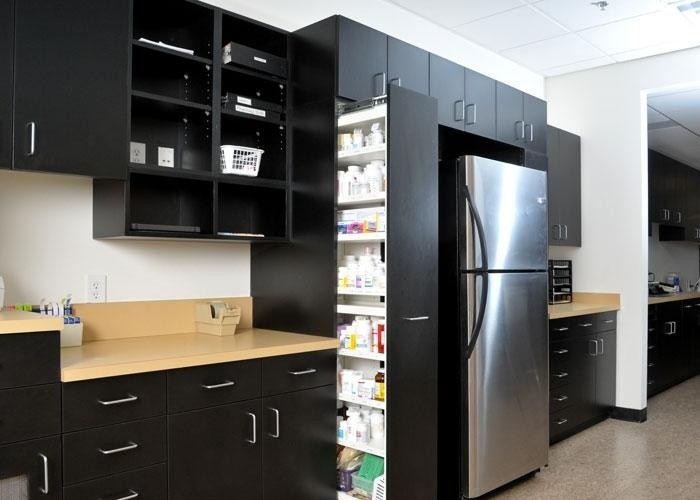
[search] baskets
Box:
[220,144,265,177]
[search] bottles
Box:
[338,121,386,444]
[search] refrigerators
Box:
[438,151,550,500]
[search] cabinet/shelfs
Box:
[549,310,617,447]
[291,11,430,102]
[334,81,445,500]
[428,52,498,142]
[168,358,262,499]
[647,298,700,399]
[523,124,581,247]
[496,82,549,154]
[0,326,60,500]
[63,371,169,500]
[648,148,700,242]
[261,347,339,500]
